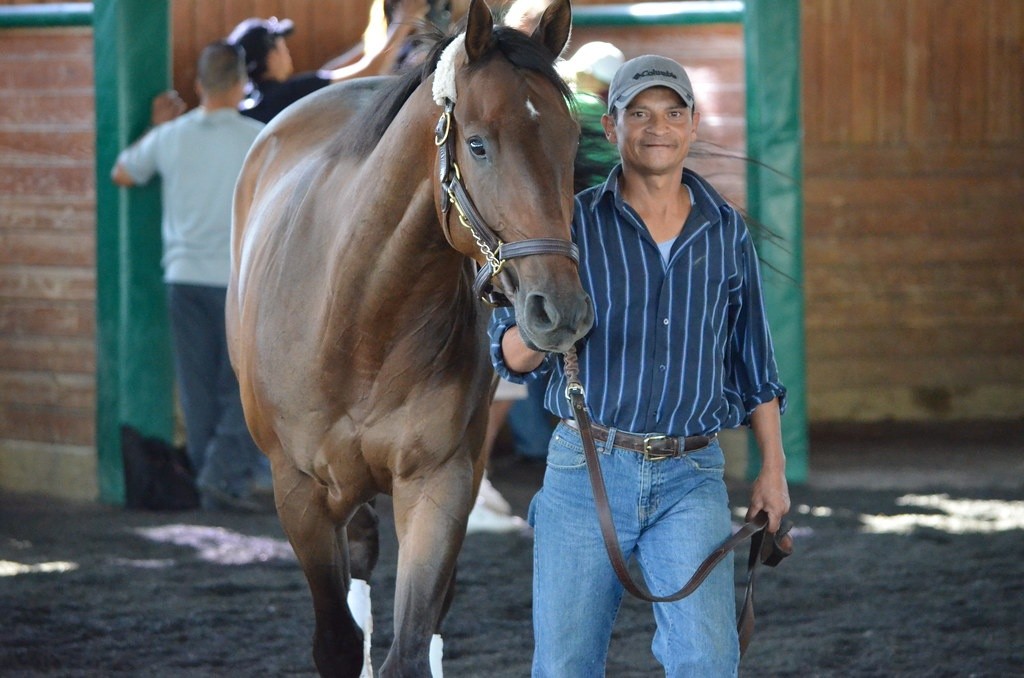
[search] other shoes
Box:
[197,466,276,514]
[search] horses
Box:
[222,0,804,678]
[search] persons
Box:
[488,53,790,676]
[112,39,274,515]
[225,0,391,124]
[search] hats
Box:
[226,17,295,72]
[606,55,695,116]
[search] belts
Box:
[562,417,718,461]
